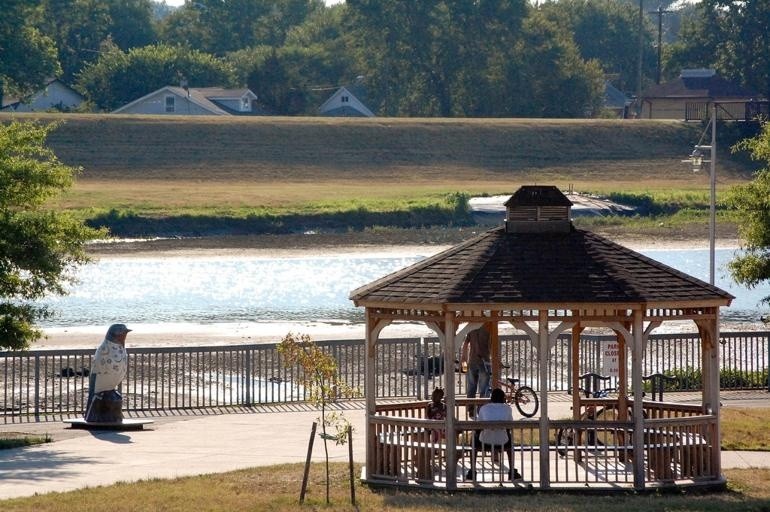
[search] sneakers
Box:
[508,469,522,480]
[465,470,477,480]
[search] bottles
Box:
[462,361,467,372]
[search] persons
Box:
[630,98,642,120]
[461,321,505,419]
[466,389,522,480]
[425,386,465,442]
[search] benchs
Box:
[379,424,706,483]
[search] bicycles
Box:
[465,359,541,419]
[594,391,647,449]
[557,385,618,455]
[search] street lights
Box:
[680,107,718,287]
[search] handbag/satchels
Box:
[483,362,492,378]
